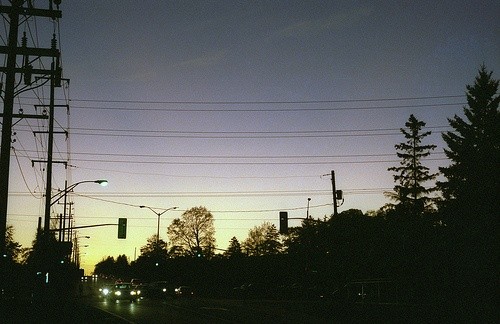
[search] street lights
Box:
[139,204,179,248]
[44,179,107,237]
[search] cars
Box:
[98,279,195,305]
